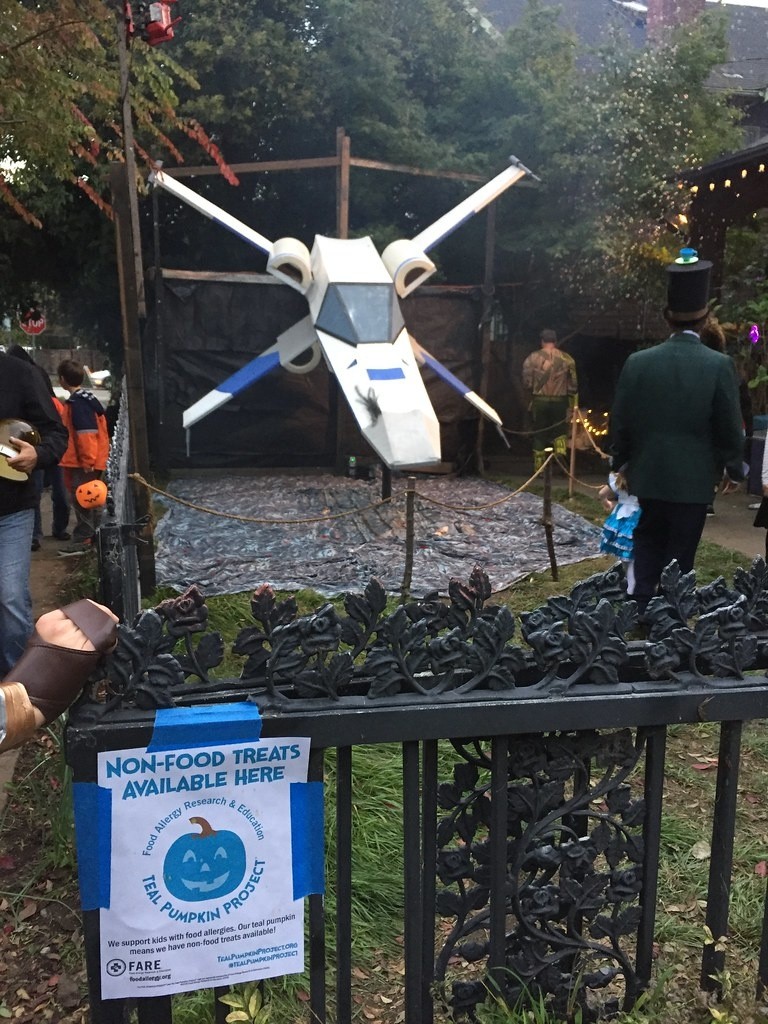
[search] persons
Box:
[0,351,70,679]
[598,464,641,601]
[612,249,746,590]
[57,360,110,554]
[754,428,768,563]
[521,331,577,475]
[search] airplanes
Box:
[148,153,543,473]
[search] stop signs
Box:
[20,309,46,335]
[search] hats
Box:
[662,247,715,321]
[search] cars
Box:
[89,370,112,389]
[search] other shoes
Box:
[706,504,715,516]
[30,539,41,550]
[52,529,71,540]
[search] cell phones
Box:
[0,442,20,458]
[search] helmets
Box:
[0,418,42,481]
